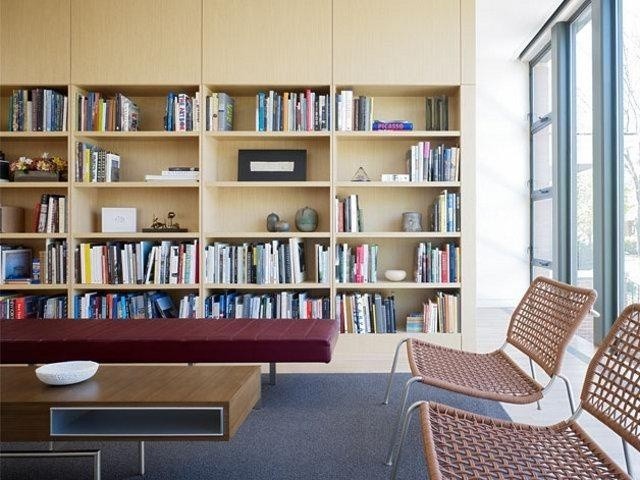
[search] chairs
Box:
[381,274,605,470]
[385,303,640,480]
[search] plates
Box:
[33,360,102,386]
[38,384,102,402]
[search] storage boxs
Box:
[96,205,138,234]
[0,203,27,234]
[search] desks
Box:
[1,366,262,480]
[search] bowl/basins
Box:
[385,269,406,282]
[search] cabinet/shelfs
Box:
[71,84,200,320]
[202,85,332,321]
[0,83,71,319]
[336,86,461,333]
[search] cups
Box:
[402,212,423,230]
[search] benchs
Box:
[0,315,341,387]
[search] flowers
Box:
[8,151,67,174]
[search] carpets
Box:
[0,372,519,480]
